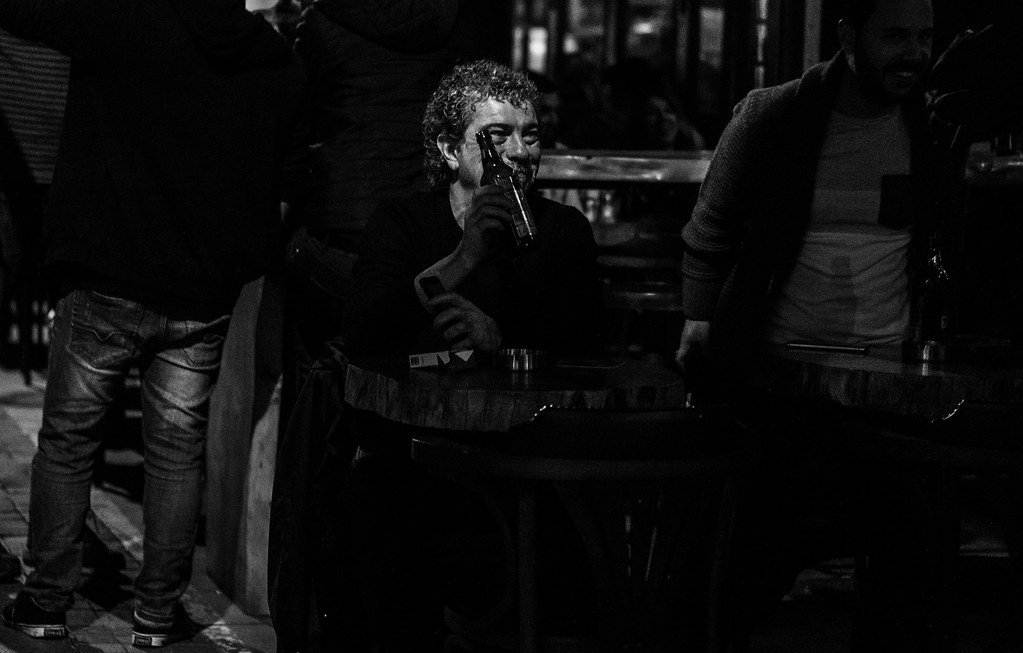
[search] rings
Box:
[465,319,474,331]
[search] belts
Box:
[286,225,345,294]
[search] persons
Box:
[0,0,306,647]
[0,0,1023,653]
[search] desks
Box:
[342,351,759,653]
[753,336,1023,653]
[603,274,708,346]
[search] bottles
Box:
[475,129,540,251]
[909,230,951,363]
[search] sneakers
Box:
[1,590,66,637]
[131,606,174,647]
